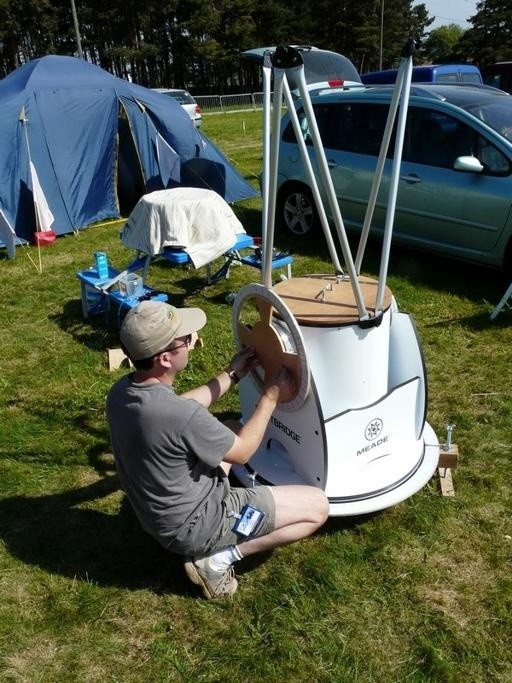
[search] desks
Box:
[119,231,255,286]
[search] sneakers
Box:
[184,551,238,601]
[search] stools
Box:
[108,284,169,329]
[76,264,124,324]
[240,251,293,280]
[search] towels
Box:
[0,209,16,258]
[27,160,55,233]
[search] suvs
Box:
[240,45,512,274]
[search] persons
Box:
[106,300,330,601]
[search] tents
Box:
[0,54,260,248]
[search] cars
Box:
[149,88,202,129]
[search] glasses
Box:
[165,333,192,352]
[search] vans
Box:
[361,63,511,94]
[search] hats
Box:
[120,299,207,361]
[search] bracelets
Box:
[225,367,240,385]
